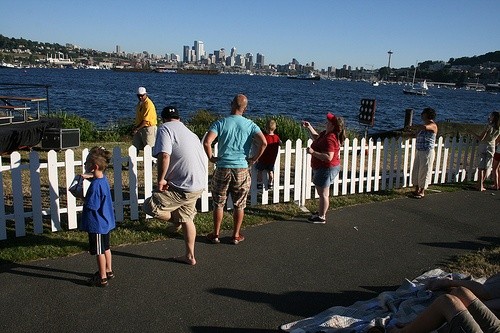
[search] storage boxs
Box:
[42,128,81,152]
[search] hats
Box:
[327,112,341,132]
[136,86,147,94]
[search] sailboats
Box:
[402,59,427,95]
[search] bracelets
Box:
[312,151,315,154]
[135,128,139,131]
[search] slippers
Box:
[414,194,425,198]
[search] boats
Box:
[284,69,321,82]
[154,66,222,76]
[370,80,381,88]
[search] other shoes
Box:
[95,270,114,278]
[207,232,220,243]
[307,214,326,223]
[231,234,244,244]
[89,277,108,286]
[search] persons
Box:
[122,87,158,170]
[468,111,500,192]
[142,106,205,266]
[252,120,282,189]
[402,108,438,198]
[78,146,116,287]
[386,286,500,333]
[204,94,268,245]
[301,114,345,224]
